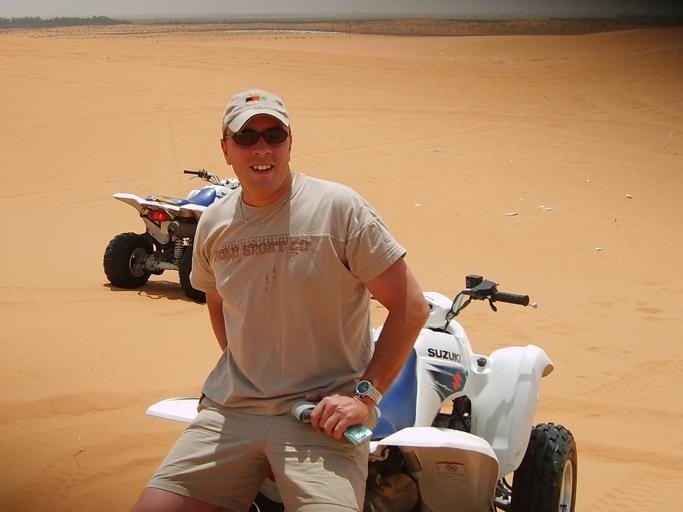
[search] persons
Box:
[128,89,431,510]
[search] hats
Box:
[220,90,290,136]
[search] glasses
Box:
[222,126,287,146]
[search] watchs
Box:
[354,380,383,405]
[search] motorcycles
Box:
[100,167,241,303]
[137,273,581,512]
